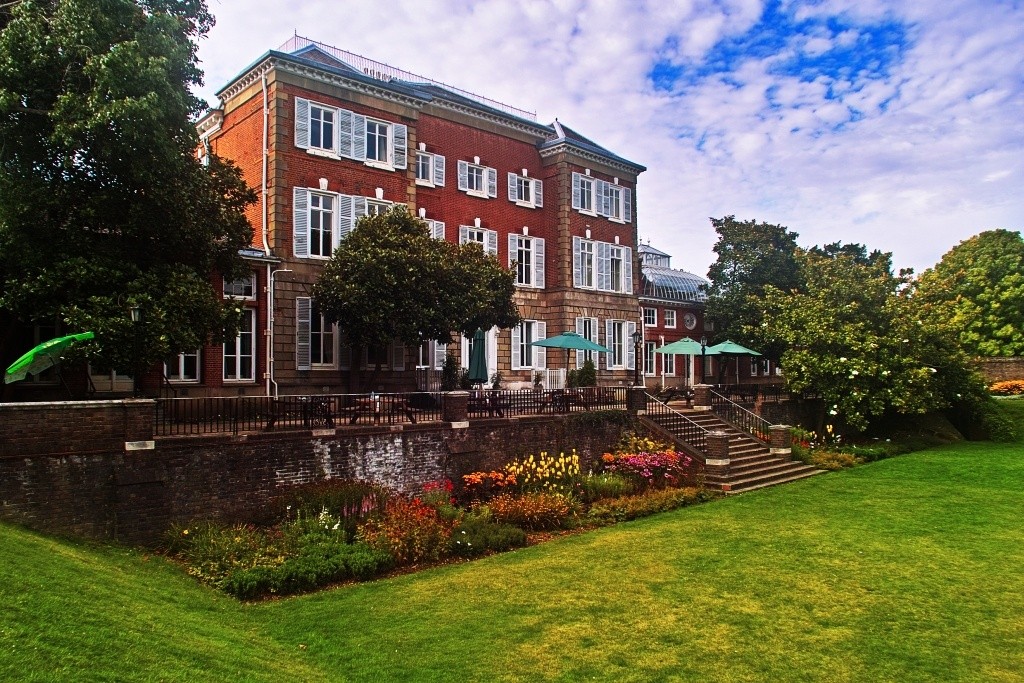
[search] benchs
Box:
[258,411,287,418]
[342,406,371,411]
[534,400,553,404]
[399,406,423,414]
[657,393,672,397]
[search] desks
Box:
[537,392,591,413]
[349,396,417,425]
[661,390,695,408]
[263,400,336,432]
[716,388,752,403]
[468,397,505,418]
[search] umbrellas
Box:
[528,331,612,382]
[650,336,722,389]
[708,339,763,390]
[4,331,97,401]
